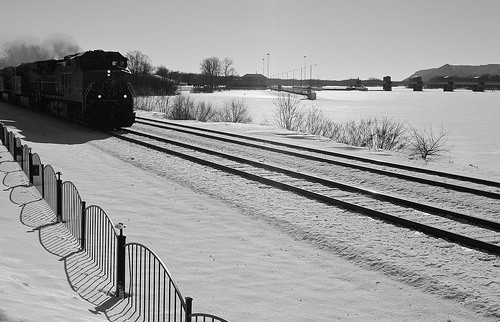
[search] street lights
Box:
[267,53,270,85]
[304,55,306,86]
[262,59,264,75]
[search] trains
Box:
[0,49,136,131]
